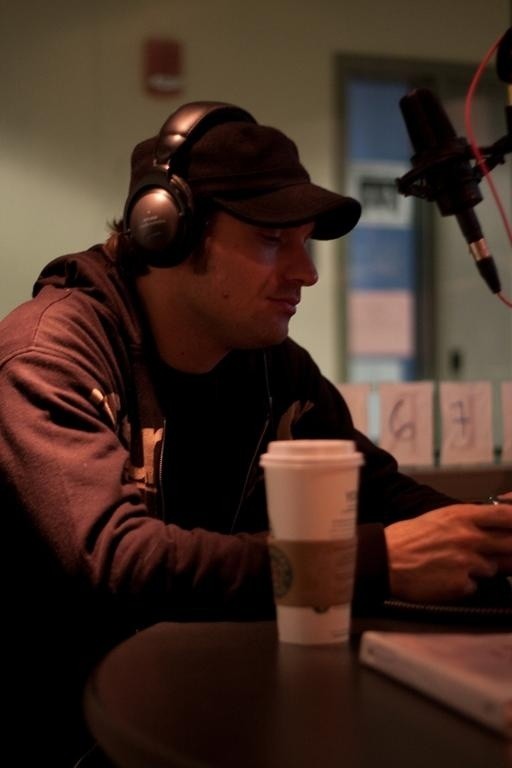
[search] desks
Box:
[81,584,512,768]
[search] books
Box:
[358,627,512,735]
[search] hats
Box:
[128,119,361,241]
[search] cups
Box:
[260,439,367,646]
[274,639,360,767]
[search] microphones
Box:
[399,87,502,293]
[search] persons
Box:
[0,101,512,767]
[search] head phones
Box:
[122,101,256,268]
